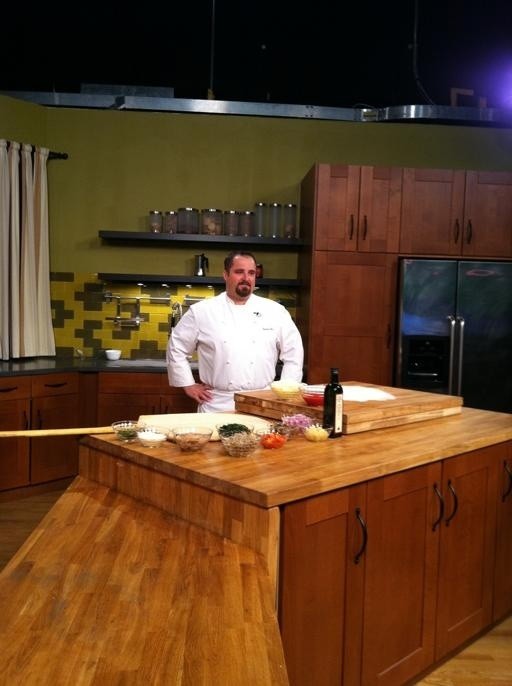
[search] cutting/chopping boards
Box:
[233,378,463,434]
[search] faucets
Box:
[172,301,184,320]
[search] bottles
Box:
[323,367,344,436]
[148,202,298,238]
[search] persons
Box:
[165,248,304,413]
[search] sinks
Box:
[106,357,200,372]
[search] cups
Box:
[194,253,209,276]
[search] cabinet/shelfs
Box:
[269,475,368,686]
[1,365,97,496]
[297,159,401,253]
[492,433,512,628]
[96,227,311,289]
[398,165,510,262]
[92,365,204,431]
[294,249,399,389]
[358,442,501,686]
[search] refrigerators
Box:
[396,255,511,413]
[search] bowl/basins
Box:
[217,421,287,458]
[299,384,326,406]
[299,423,332,442]
[136,425,171,448]
[112,420,148,444]
[268,379,308,400]
[171,426,214,452]
[105,349,122,361]
